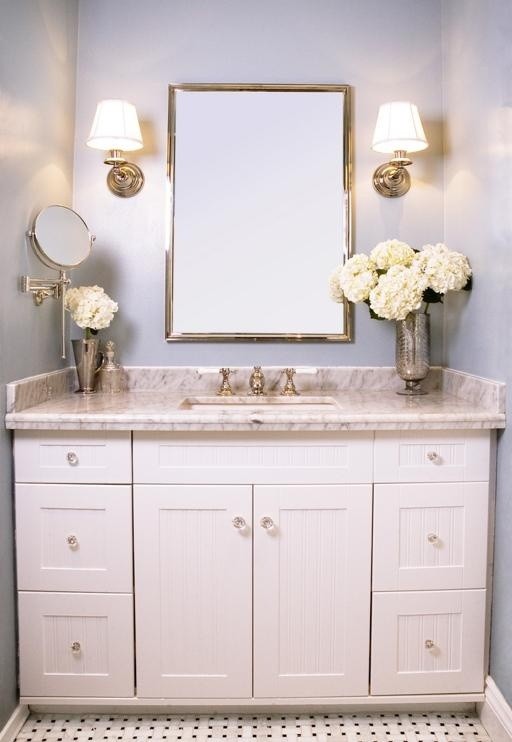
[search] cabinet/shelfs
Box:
[12,431,490,707]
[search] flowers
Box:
[64,285,119,339]
[338,239,472,322]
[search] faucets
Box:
[248,366,266,395]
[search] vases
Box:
[395,301,431,396]
[71,338,103,394]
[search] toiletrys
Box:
[99,341,124,394]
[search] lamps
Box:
[86,99,145,198]
[370,101,429,198]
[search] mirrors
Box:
[32,205,92,267]
[164,83,354,345]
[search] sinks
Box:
[176,395,345,414]
